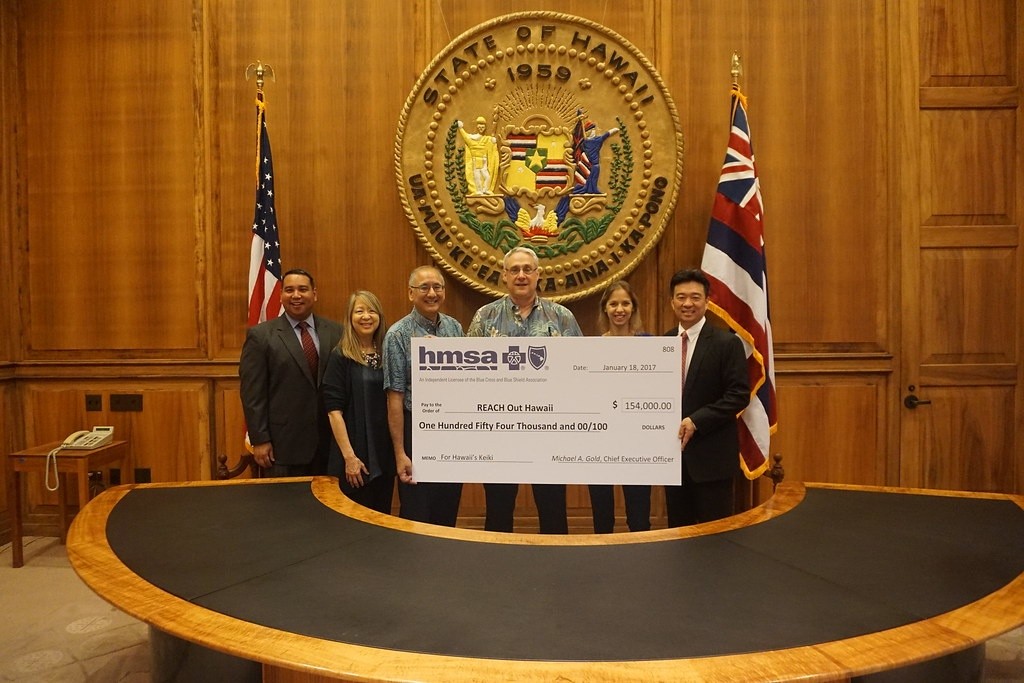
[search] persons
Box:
[588,280,652,534]
[662,268,750,531]
[467,247,583,535]
[382,266,464,528]
[321,290,395,515]
[237,269,344,477]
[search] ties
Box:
[299,322,318,385]
[681,331,688,389]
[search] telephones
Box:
[61,426,114,450]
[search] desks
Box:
[7,440,129,567]
[64,473,1024,683]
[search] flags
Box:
[244,90,286,453]
[700,89,778,480]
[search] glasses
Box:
[506,267,538,275]
[409,284,445,293]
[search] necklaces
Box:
[362,339,381,370]
